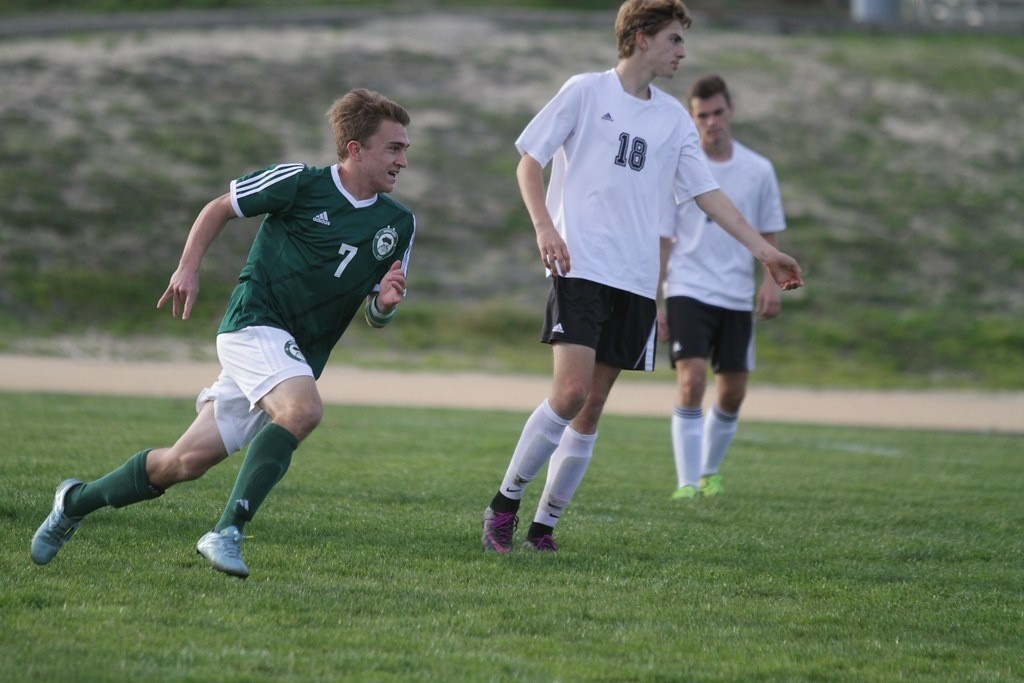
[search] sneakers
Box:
[196,525,250,579]
[481,506,520,555]
[522,535,558,553]
[30,478,87,565]
[700,473,724,498]
[672,485,697,501]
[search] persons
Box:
[654,76,789,500]
[481,1,804,552]
[30,88,418,580]
